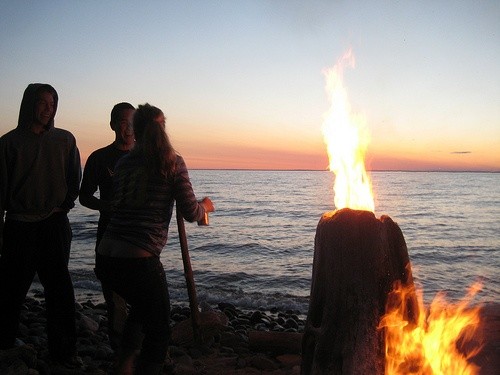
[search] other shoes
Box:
[59,356,83,369]
[161,350,176,372]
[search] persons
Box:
[0,83,82,375]
[78,103,214,375]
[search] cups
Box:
[198,200,209,226]
